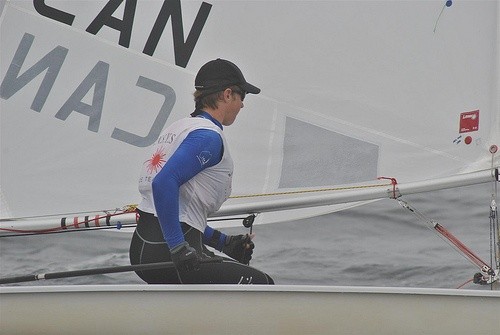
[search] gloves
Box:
[172,240,204,273]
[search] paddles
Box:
[1,257,222,285]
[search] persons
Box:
[129,58,274,287]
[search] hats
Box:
[197,60,262,95]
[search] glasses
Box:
[231,88,247,100]
